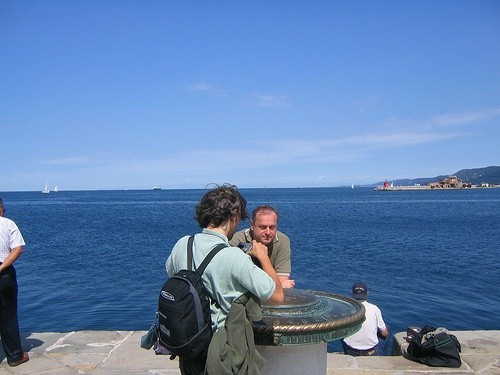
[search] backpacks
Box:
[156,235,228,360]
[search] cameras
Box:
[237,242,252,254]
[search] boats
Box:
[153,186,161,191]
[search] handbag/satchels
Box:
[404,327,462,368]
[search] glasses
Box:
[0,206,6,212]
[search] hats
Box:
[353,283,368,300]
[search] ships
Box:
[374,175,500,191]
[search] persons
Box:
[228,206,295,288]
[165,183,284,375]
[340,282,394,356]
[0,198,29,367]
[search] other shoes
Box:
[9,352,29,365]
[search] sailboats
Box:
[53,186,60,193]
[42,181,49,194]
